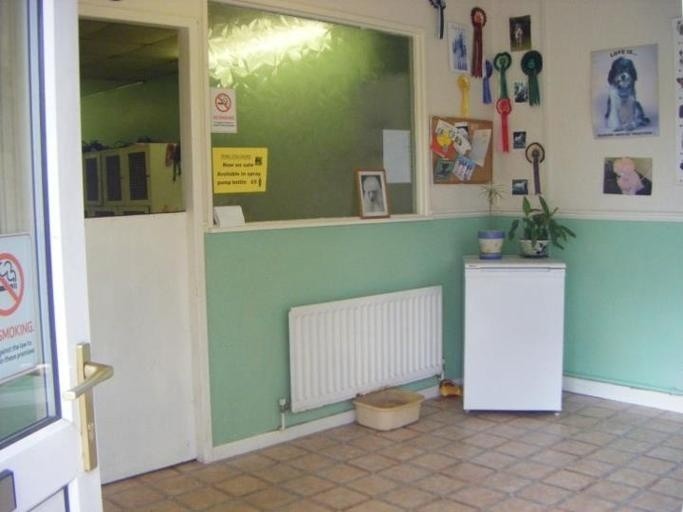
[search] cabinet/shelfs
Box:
[462,254,566,414]
[82,142,185,219]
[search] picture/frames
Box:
[353,168,390,221]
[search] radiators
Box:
[283,282,445,417]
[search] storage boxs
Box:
[352,390,421,429]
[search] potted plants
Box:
[469,182,508,260]
[508,196,575,256]
